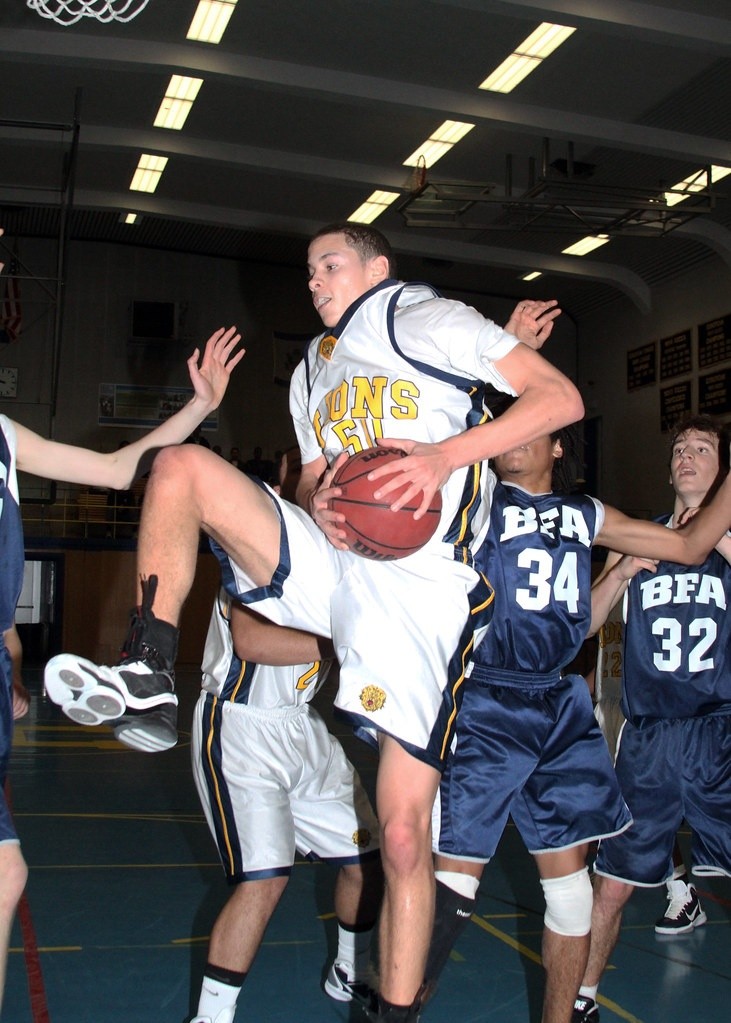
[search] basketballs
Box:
[326,446,443,561]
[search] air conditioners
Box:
[127,299,179,342]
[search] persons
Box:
[572,413,731,1023]
[44,220,588,1023]
[184,445,384,1023]
[422,299,731,1023]
[0,320,248,1023]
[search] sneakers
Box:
[44,572,179,752]
[190,1004,237,1023]
[325,958,380,1012]
[572,994,600,1023]
[654,880,707,935]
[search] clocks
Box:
[0,366,19,399]
